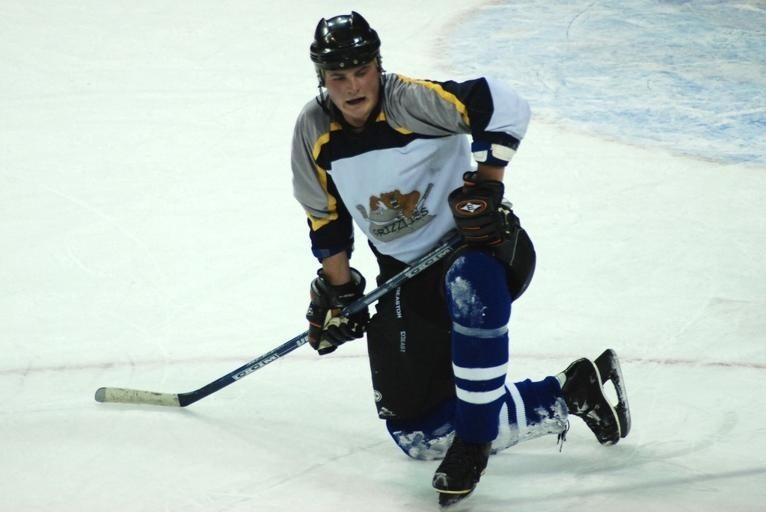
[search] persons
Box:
[290,10,621,496]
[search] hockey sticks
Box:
[94,198,513,407]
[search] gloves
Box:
[447,171,508,249]
[305,267,370,355]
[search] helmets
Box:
[310,11,381,69]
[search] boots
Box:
[431,437,494,495]
[554,358,622,447]
